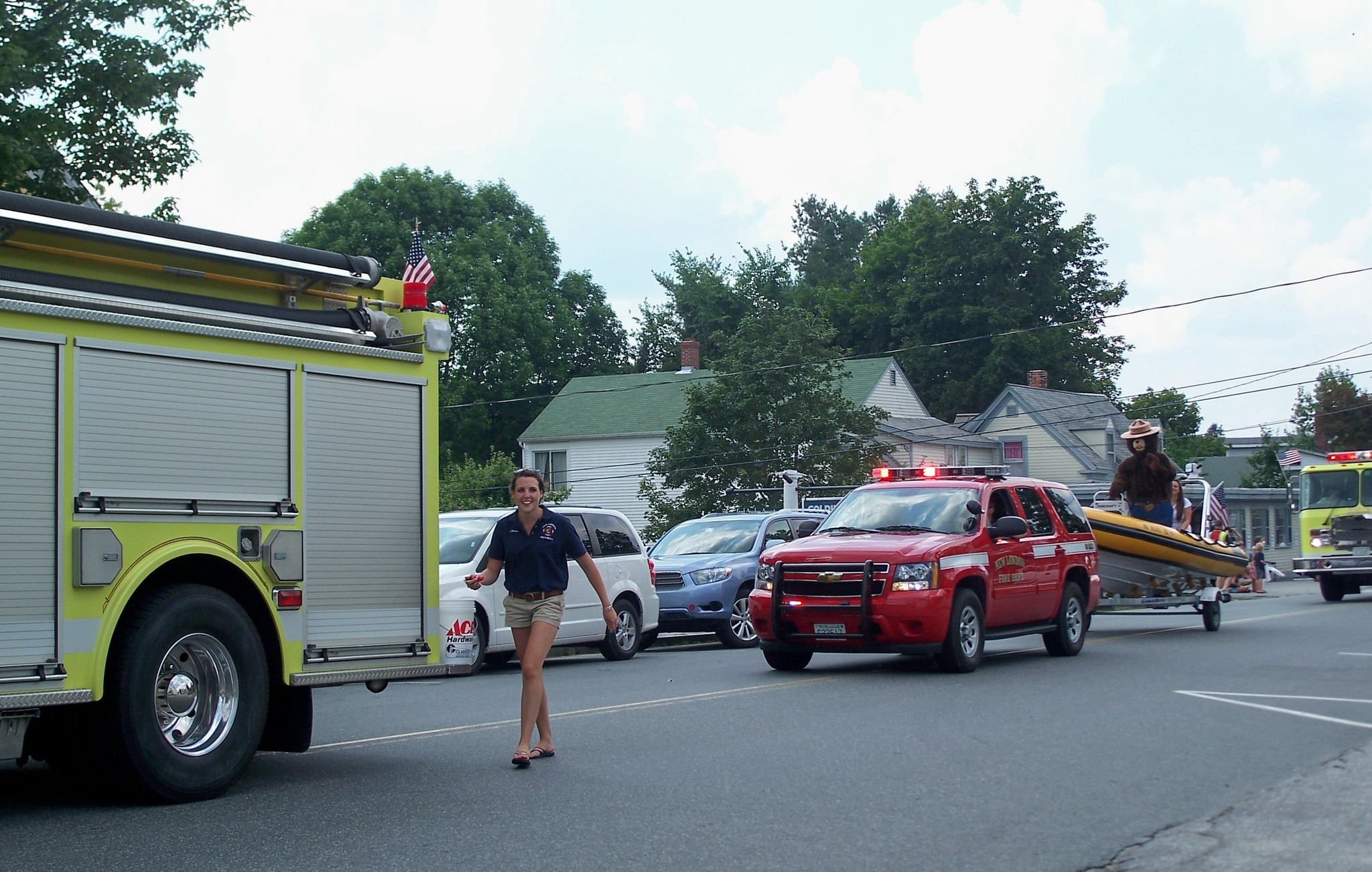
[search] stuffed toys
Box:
[1110,419,1176,529]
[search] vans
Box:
[437,501,660,677]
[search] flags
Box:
[1277,448,1301,466]
[1209,483,1229,530]
[402,227,435,289]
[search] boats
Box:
[1081,463,1249,597]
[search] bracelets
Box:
[601,604,613,611]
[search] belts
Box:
[1131,499,1171,510]
[509,589,563,602]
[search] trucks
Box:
[0,184,451,805]
[1286,450,1372,602]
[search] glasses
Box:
[513,468,540,477]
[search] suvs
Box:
[747,465,1100,672]
[644,509,833,650]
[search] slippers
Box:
[512,750,530,765]
[529,747,555,759]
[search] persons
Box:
[1224,525,1245,588]
[1170,480,1193,534]
[1250,535,1268,594]
[1237,541,1252,580]
[986,498,996,528]
[1209,519,1235,592]
[1315,486,1346,504]
[462,468,620,766]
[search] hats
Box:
[1120,419,1161,439]
[1215,519,1222,526]
